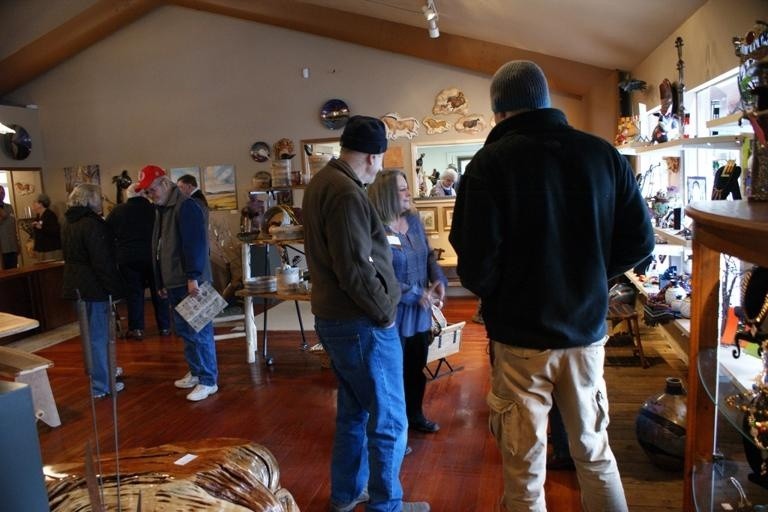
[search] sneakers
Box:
[174,371,200,388]
[186,384,218,401]
[328,483,431,512]
[92,367,125,398]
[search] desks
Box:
[0,312,40,339]
[1,381,50,512]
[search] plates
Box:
[243,275,276,294]
[275,266,299,295]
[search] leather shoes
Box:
[408,415,440,431]
[120,329,171,340]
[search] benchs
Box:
[1,346,62,429]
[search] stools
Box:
[606,302,652,370]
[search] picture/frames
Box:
[415,207,439,235]
[442,207,455,231]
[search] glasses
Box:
[144,179,167,194]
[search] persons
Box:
[61,184,124,399]
[297,114,433,512]
[105,167,210,338]
[134,165,219,401]
[547,396,575,471]
[446,59,656,512]
[0,186,20,270]
[27,194,64,261]
[366,164,448,456]
[415,159,463,199]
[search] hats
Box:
[490,60,550,111]
[340,115,387,154]
[135,165,165,193]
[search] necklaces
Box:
[710,166,735,201]
[724,339,768,480]
[741,270,768,324]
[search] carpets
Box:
[39,437,301,512]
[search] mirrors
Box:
[300,136,341,186]
[409,139,486,204]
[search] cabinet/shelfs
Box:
[0,262,81,352]
[240,206,332,370]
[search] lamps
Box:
[422,0,442,39]
